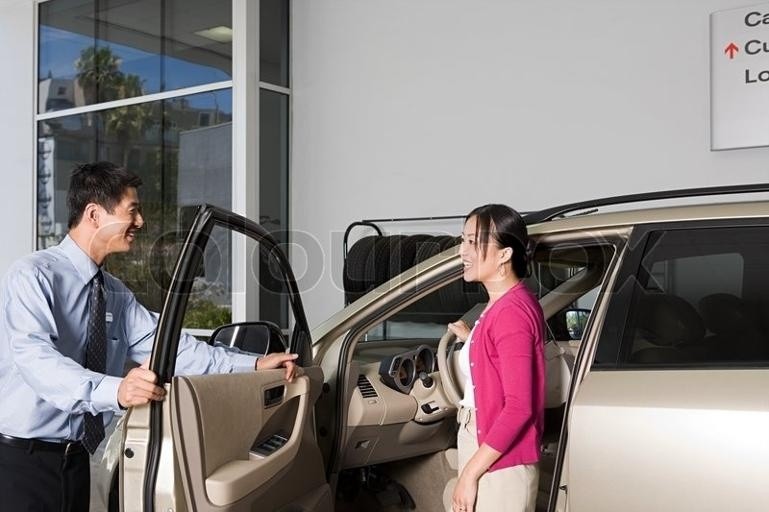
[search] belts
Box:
[457,406,477,424]
[0,433,85,456]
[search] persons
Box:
[448,204,547,512]
[0,160,301,512]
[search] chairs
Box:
[698,293,769,363]
[536,294,707,512]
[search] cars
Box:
[98,183,769,512]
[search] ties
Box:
[80,270,105,455]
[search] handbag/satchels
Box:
[543,319,572,409]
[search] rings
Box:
[458,505,466,511]
